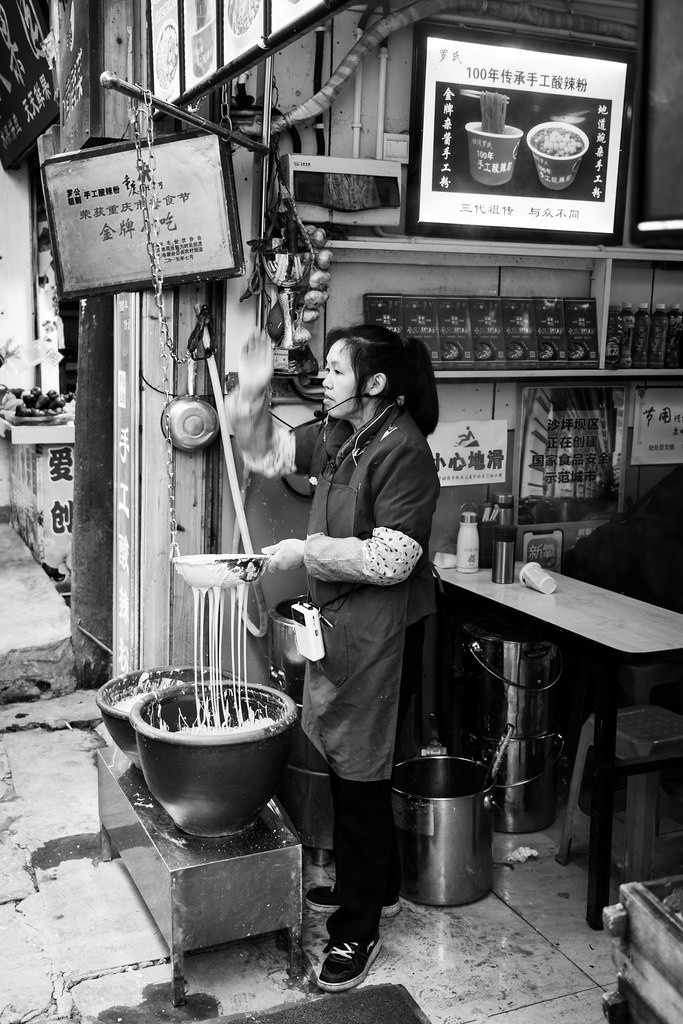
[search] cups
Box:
[519,562,558,594]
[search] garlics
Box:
[293,224,333,344]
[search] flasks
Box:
[492,524,518,584]
[494,493,514,526]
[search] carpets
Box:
[187,982,435,1024]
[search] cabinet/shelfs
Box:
[321,240,683,382]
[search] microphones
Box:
[314,393,372,419]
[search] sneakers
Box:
[305,885,401,918]
[317,931,382,993]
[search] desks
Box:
[428,560,683,930]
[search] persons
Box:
[229,325,439,991]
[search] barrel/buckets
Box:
[456,619,570,834]
[392,754,496,907]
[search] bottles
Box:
[606,300,683,372]
[455,511,479,573]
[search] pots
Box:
[159,345,221,451]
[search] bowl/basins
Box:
[526,121,589,190]
[465,121,524,186]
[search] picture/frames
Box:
[404,18,639,246]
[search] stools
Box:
[555,664,683,884]
